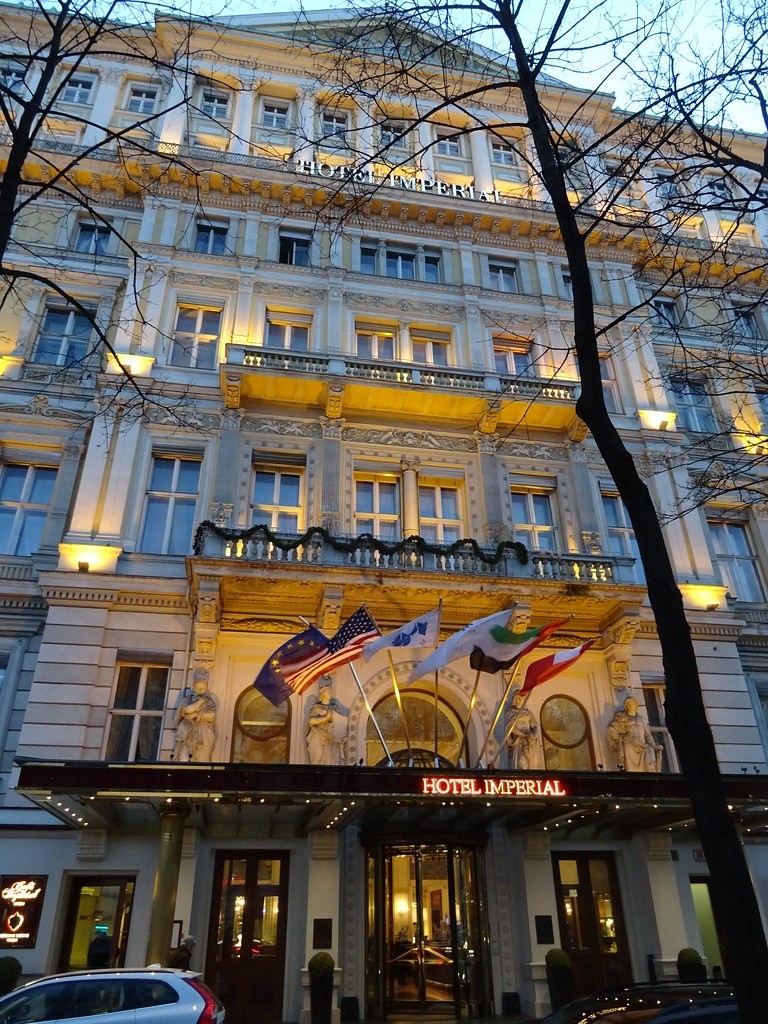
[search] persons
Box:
[169,934,197,970]
[606,696,665,772]
[305,684,348,764]
[86,929,120,970]
[504,689,539,770]
[394,925,412,986]
[170,677,217,763]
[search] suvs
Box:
[0,967,225,1024]
[537,980,740,1024]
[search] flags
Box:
[252,624,330,706]
[517,634,603,697]
[408,609,514,685]
[468,617,570,676]
[361,609,439,664]
[278,604,383,696]
